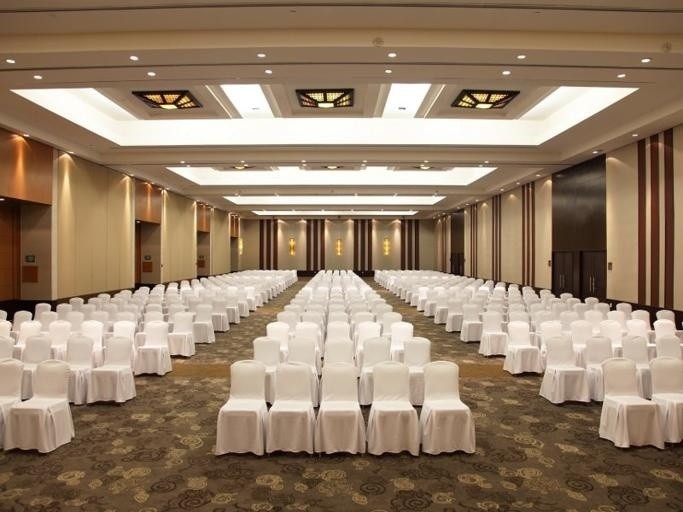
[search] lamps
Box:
[382,237,391,257]
[295,88,354,109]
[335,238,342,256]
[288,238,296,257]
[131,90,203,109]
[450,89,520,109]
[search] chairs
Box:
[216,268,476,456]
[373,269,683,457]
[1,268,299,455]
